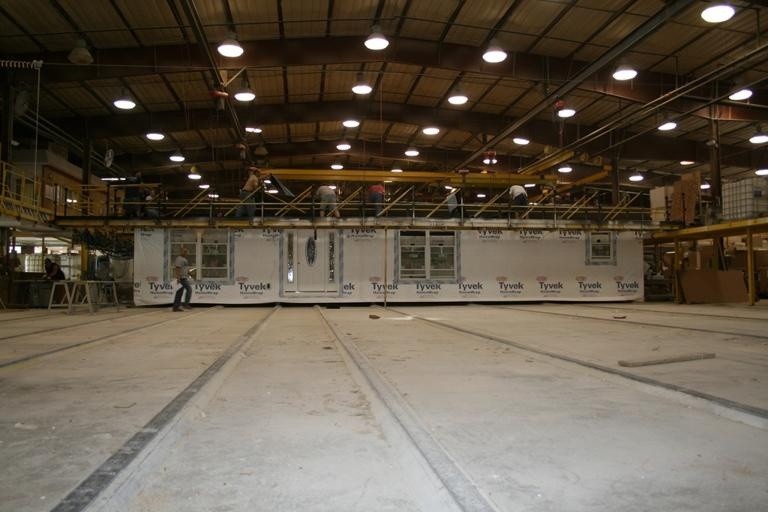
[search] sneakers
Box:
[173,304,191,311]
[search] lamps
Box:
[216,31,263,136]
[513,54,639,147]
[329,23,390,171]
[404,36,508,158]
[113,85,220,200]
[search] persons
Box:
[171,247,200,311]
[316,183,340,217]
[368,182,386,217]
[44,257,65,303]
[509,184,529,219]
[235,169,264,219]
